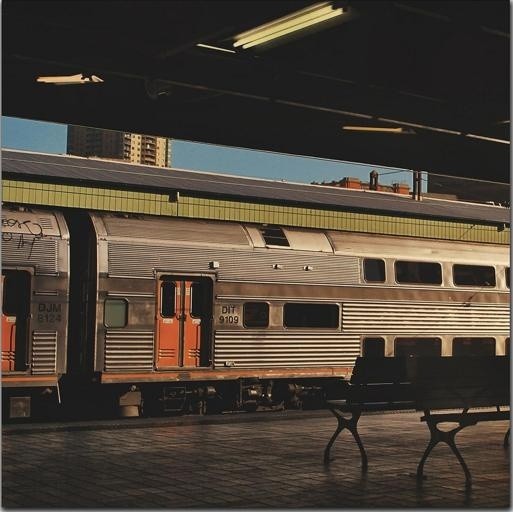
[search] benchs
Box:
[322,356,414,473]
[414,356,511,487]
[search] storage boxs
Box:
[341,125,418,135]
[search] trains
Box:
[1,147,509,418]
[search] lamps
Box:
[232,1,346,50]
[32,74,105,87]
